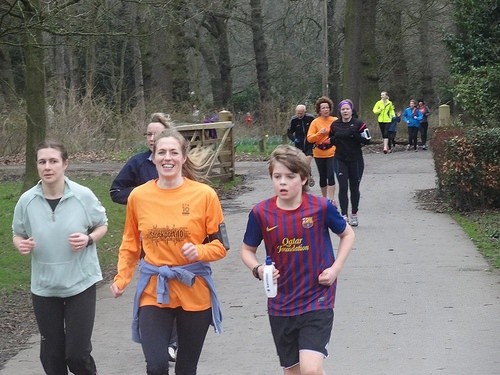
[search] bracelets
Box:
[86,234,93,247]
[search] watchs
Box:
[252,263,262,280]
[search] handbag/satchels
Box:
[314,143,335,150]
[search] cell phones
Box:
[219,223,229,250]
[364,129,371,140]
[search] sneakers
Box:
[348,211,359,226]
[341,215,349,223]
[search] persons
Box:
[12,141,108,375]
[240,143,355,375]
[307,96,339,206]
[372,91,402,154]
[110,113,212,362]
[417,99,432,150]
[244,112,254,128]
[192,104,200,116]
[286,104,316,192]
[402,100,424,152]
[328,99,372,227]
[110,129,230,375]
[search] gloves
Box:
[294,138,301,143]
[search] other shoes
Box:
[388,149,391,153]
[331,200,335,205]
[168,343,178,362]
[383,149,387,154]
[422,144,426,150]
[414,146,418,152]
